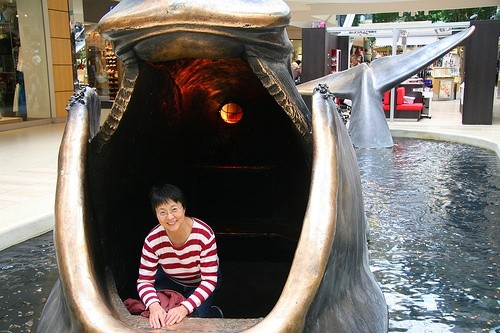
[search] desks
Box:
[399,83,433,118]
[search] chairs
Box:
[396,86,424,120]
[381,90,390,116]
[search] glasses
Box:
[156,206,183,215]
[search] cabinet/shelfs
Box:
[331,49,341,107]
[97,48,120,100]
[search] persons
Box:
[138,182,219,329]
